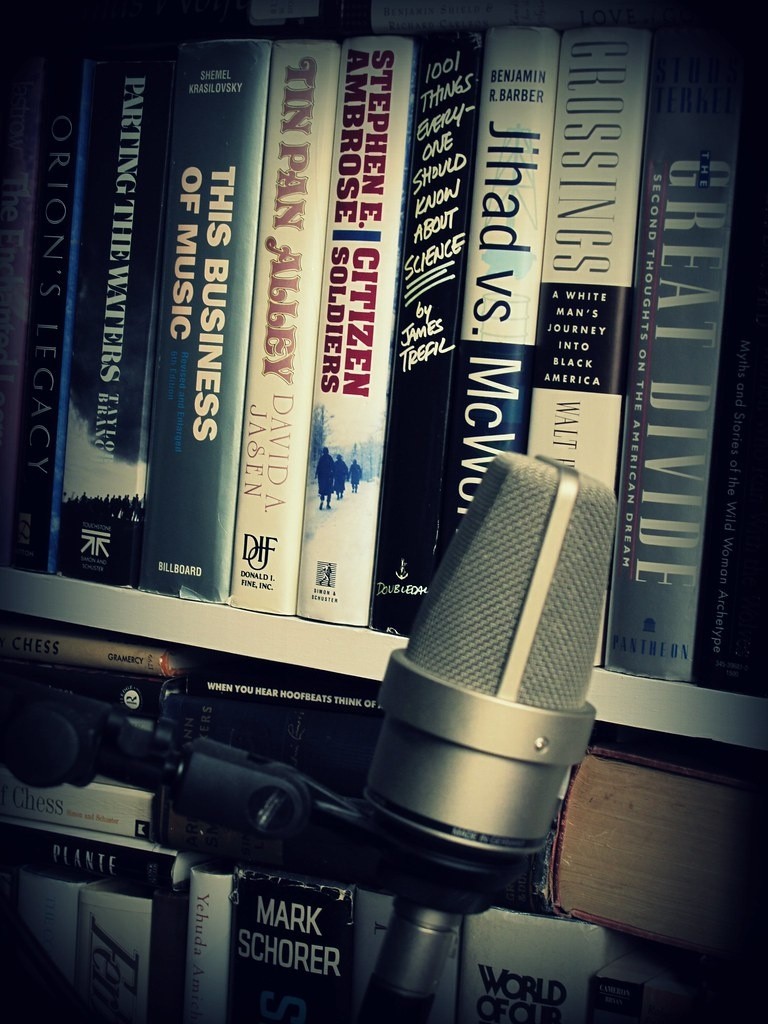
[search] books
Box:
[0,0,768,1024]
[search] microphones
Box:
[354,450,616,1024]
